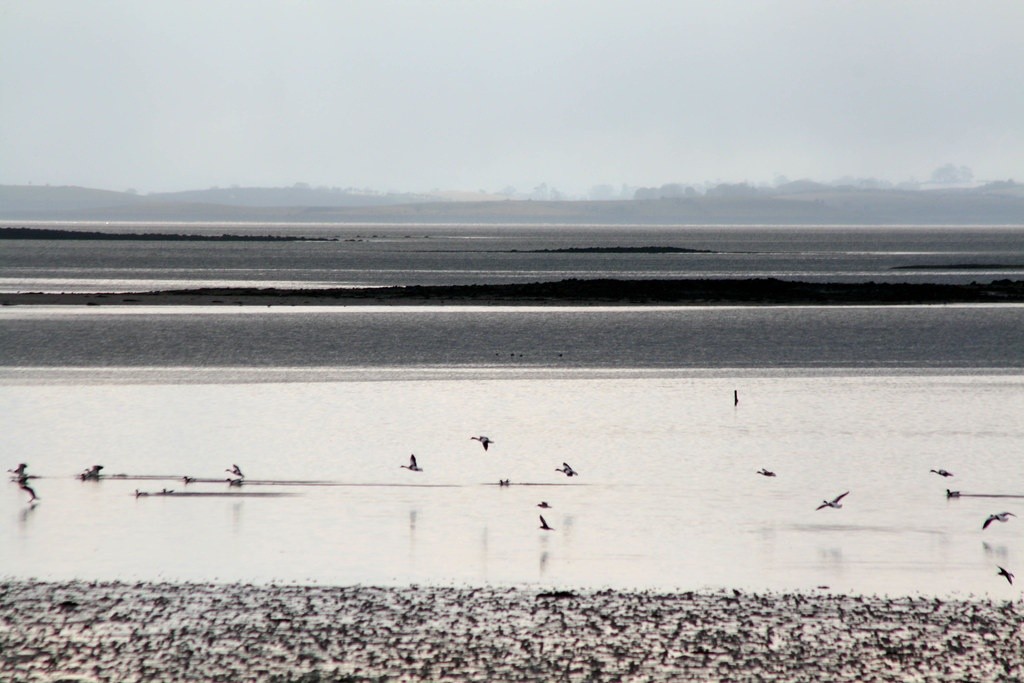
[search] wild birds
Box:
[183,476,195,484]
[997,565,1014,585]
[471,436,494,451]
[816,491,849,511]
[135,488,174,498]
[81,466,103,481]
[7,463,39,502]
[946,488,960,497]
[757,467,775,477]
[537,501,553,529]
[555,462,579,477]
[930,469,952,477]
[225,464,245,486]
[401,454,422,471]
[983,512,1018,529]
